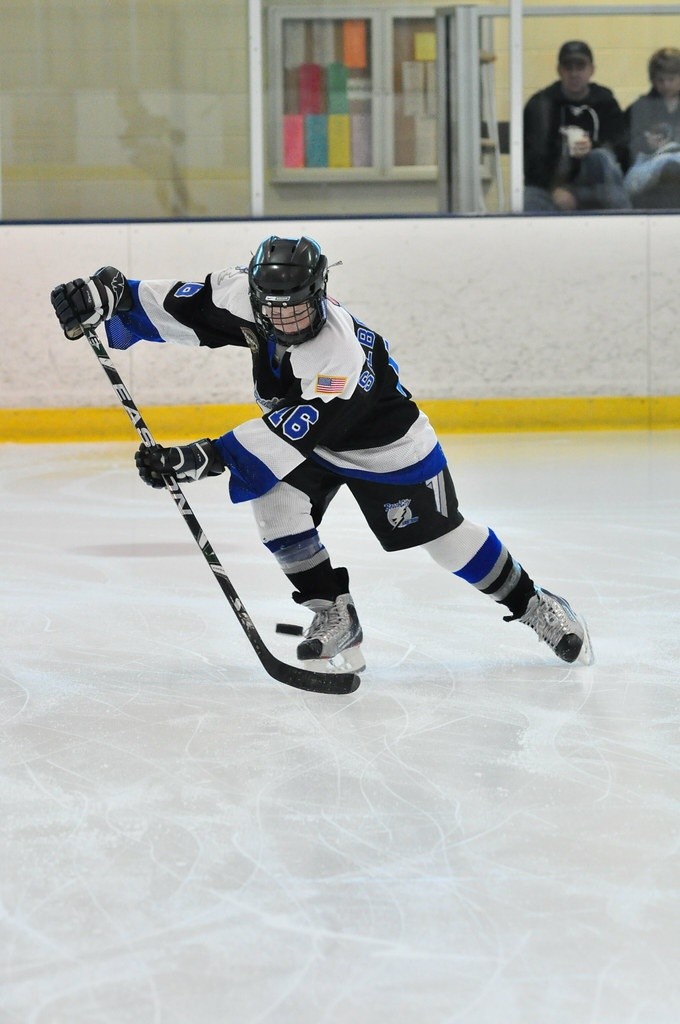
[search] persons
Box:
[51,236,596,675]
[524,41,680,211]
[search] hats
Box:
[559,42,593,64]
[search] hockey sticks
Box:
[80,322,362,695]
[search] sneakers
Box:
[496,563,594,668]
[275,566,367,674]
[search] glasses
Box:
[561,62,588,71]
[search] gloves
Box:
[133,436,226,489]
[49,267,132,341]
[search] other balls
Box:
[275,623,303,636]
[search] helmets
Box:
[248,236,329,344]
